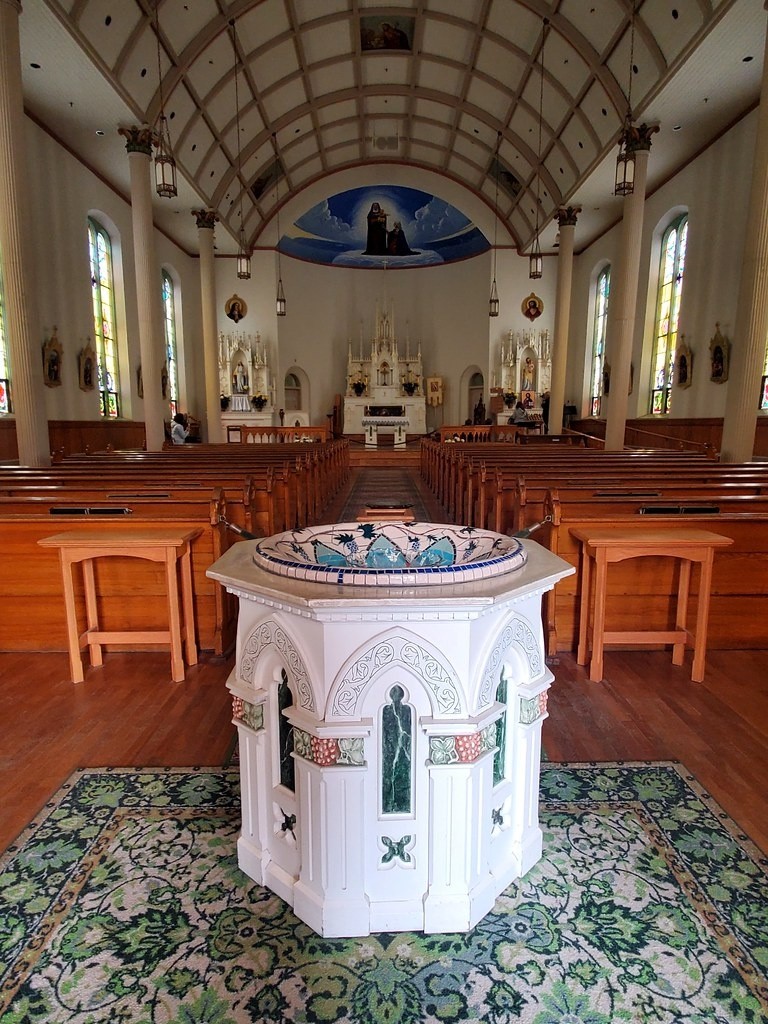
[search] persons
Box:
[366,203,420,256]
[229,303,243,320]
[523,393,533,409]
[524,357,535,391]
[542,392,566,429]
[171,412,190,444]
[508,402,528,424]
[233,360,246,393]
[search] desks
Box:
[36,527,205,683]
[361,416,410,449]
[568,527,734,683]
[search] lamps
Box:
[154,0,286,317]
[489,0,636,317]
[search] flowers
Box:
[402,382,418,392]
[250,395,268,408]
[353,382,367,394]
[220,392,233,409]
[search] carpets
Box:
[0,760,768,1023]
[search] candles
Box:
[402,404,404,410]
[367,404,369,410]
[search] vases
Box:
[357,392,361,396]
[221,408,226,412]
[408,392,413,396]
[256,408,262,412]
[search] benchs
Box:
[419,436,768,657]
[0,439,350,658]
